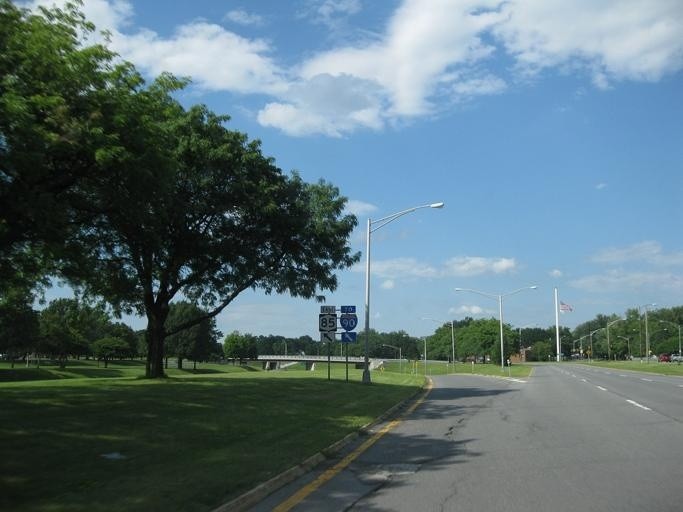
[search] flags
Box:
[560,301,573,312]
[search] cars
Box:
[465,355,492,364]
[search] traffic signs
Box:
[340,314,358,330]
[321,305,336,314]
[341,305,357,314]
[318,314,338,332]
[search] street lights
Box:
[381,343,403,377]
[402,333,428,377]
[453,285,538,370]
[422,316,457,375]
[557,302,683,366]
[362,202,450,384]
